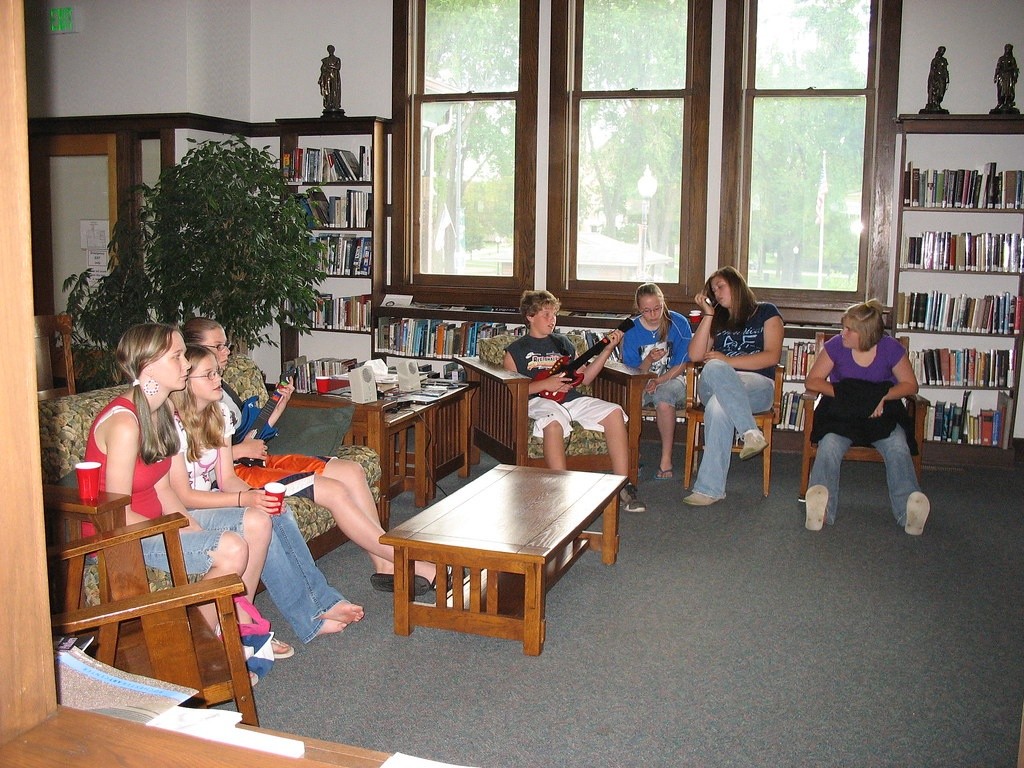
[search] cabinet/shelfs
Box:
[891,114,1024,469]
[274,116,389,399]
[372,308,817,455]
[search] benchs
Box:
[35,355,380,612]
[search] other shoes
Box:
[805,485,828,532]
[370,574,430,595]
[430,566,454,591]
[740,433,769,460]
[683,494,722,505]
[905,492,930,536]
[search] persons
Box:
[994,44,1019,109]
[928,46,949,108]
[169,343,365,644]
[622,283,692,480]
[82,323,295,686]
[504,290,646,512]
[683,265,784,505]
[183,317,454,596]
[320,45,341,110]
[805,298,930,535]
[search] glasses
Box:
[203,342,230,350]
[187,369,223,380]
[639,305,663,314]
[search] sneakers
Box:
[619,482,645,512]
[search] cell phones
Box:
[705,298,712,306]
[657,348,664,351]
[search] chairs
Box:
[34,314,75,404]
[45,511,259,728]
[640,361,786,498]
[798,331,930,503]
[450,332,659,489]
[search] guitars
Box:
[232,364,298,468]
[532,317,636,404]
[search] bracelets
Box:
[238,491,242,507]
[704,314,714,316]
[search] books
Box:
[375,317,526,359]
[903,230,1024,273]
[418,362,465,381]
[283,293,371,332]
[895,336,1016,388]
[903,161,1024,210]
[780,342,815,381]
[776,390,805,431]
[283,146,371,182]
[294,187,372,228]
[308,233,371,275]
[284,356,365,393]
[924,390,1014,450]
[568,329,620,362]
[896,292,1022,334]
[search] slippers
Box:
[655,464,674,479]
[270,637,294,658]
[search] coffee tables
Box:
[377,464,628,656]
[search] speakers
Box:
[396,361,421,392]
[349,366,377,404]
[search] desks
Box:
[233,721,398,768]
[318,379,479,508]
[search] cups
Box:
[690,310,701,321]
[689,313,700,322]
[264,483,286,514]
[74,462,102,500]
[315,376,330,394]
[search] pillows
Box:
[265,405,356,457]
